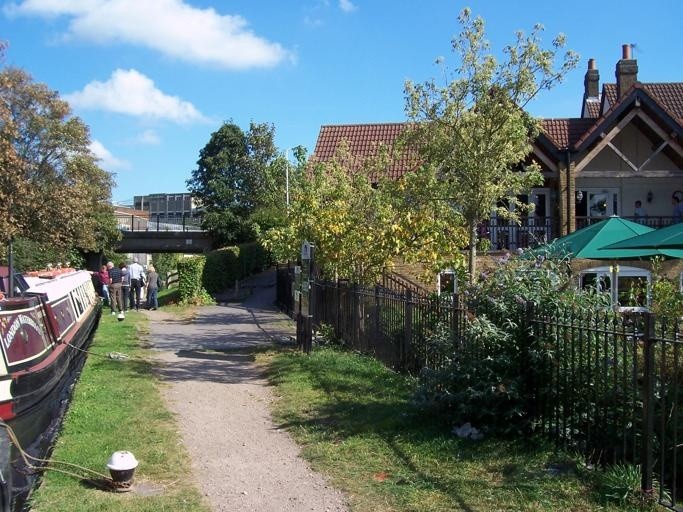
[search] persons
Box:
[86,257,160,316]
[670,196,682,224]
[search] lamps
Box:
[576,190,583,203]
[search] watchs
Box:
[633,199,649,224]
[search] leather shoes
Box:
[124,307,157,311]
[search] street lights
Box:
[284,143,305,215]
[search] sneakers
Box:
[112,312,115,316]
[120,311,124,314]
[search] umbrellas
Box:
[506,216,682,314]
[597,222,682,251]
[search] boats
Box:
[0,233,107,426]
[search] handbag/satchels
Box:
[140,277,145,288]
[157,277,163,289]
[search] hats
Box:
[146,266,155,272]
[132,257,138,262]
